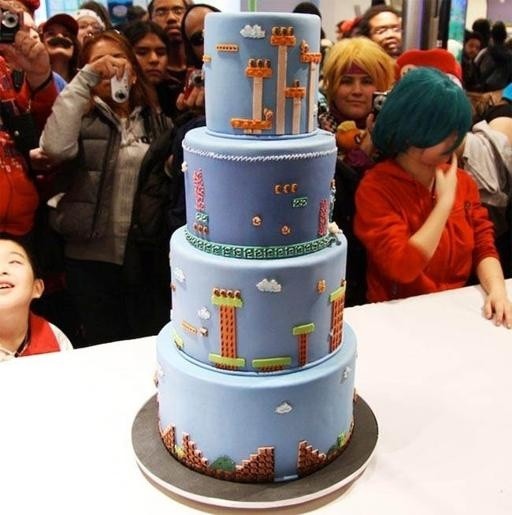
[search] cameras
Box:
[111,66,130,103]
[184,67,204,101]
[0,8,24,43]
[371,92,388,121]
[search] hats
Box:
[393,47,462,83]
[43,14,78,37]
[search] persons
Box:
[0,0,512,362]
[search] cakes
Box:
[154,11,359,485]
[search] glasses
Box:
[186,33,203,47]
[152,7,185,15]
